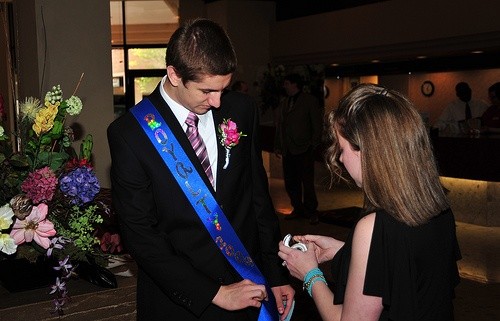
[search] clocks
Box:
[422,81,434,96]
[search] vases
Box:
[0,254,61,294]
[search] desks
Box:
[0,260,137,321]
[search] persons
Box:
[277,85,462,321]
[481,81,500,133]
[432,83,488,129]
[231,80,251,97]
[280,73,322,222]
[107,20,296,321]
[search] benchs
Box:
[439,177,500,285]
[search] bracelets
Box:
[302,267,327,296]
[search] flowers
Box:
[0,72,128,314]
[218,117,247,169]
